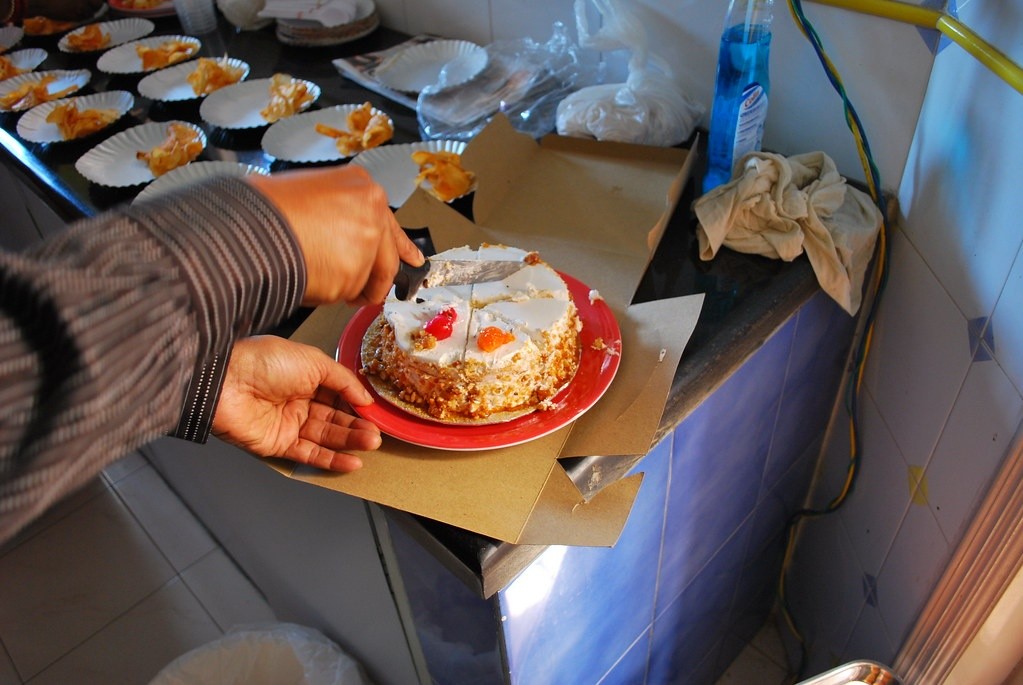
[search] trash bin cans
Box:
[795,659,905,685]
[150,629,364,685]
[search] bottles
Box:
[702,0,775,197]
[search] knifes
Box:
[394,256,532,302]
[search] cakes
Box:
[359,242,584,421]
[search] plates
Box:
[376,40,488,91]
[347,139,476,209]
[58,17,154,54]
[3,48,48,70]
[75,120,206,188]
[108,0,176,17]
[276,0,380,46]
[16,90,135,144]
[334,270,622,451]
[0,69,91,113]
[0,27,24,52]
[96,35,201,73]
[198,76,321,130]
[261,103,394,162]
[130,160,272,207]
[137,58,251,102]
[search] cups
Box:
[172,0,217,36]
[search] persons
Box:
[0,165,425,547]
